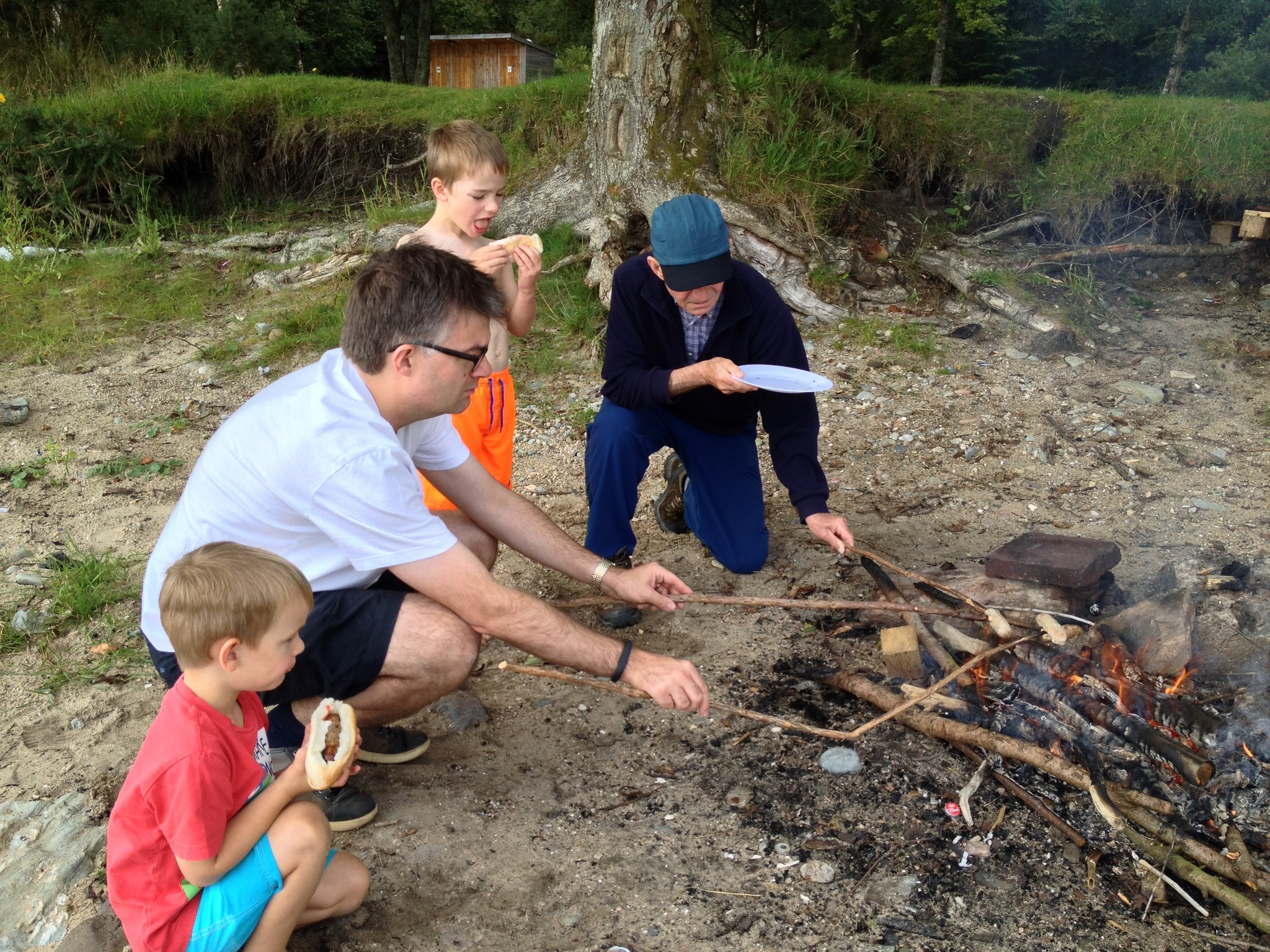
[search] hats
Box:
[651,195,734,291]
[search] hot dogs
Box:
[1041,624,1082,642]
[1036,613,1066,646]
[489,233,544,262]
[984,608,1012,638]
[305,697,356,791]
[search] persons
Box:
[140,243,710,831]
[107,541,370,952]
[395,120,542,640]
[584,194,855,629]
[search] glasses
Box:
[387,341,488,373]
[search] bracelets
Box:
[591,557,616,589]
[611,639,633,682]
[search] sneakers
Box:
[349,721,430,763]
[654,453,690,534]
[266,746,381,830]
[595,547,643,629]
